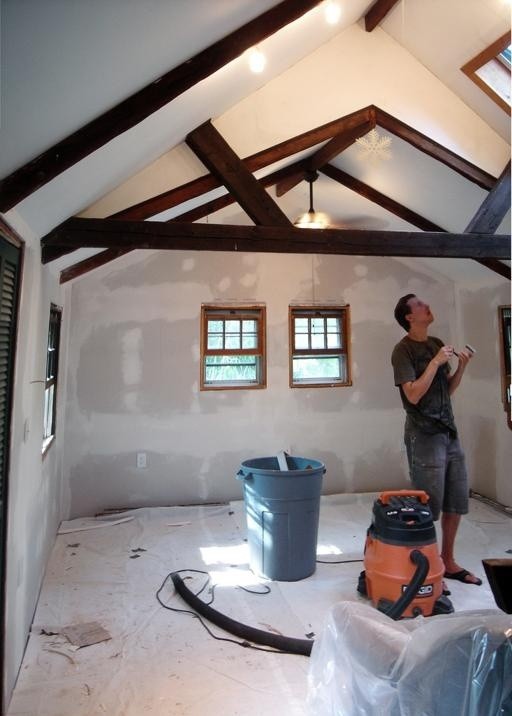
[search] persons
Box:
[392,294,482,587]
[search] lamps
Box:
[295,170,334,229]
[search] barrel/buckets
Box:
[234,455,328,583]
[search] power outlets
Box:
[136,453,146,469]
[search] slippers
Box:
[443,569,482,586]
[442,581,451,596]
